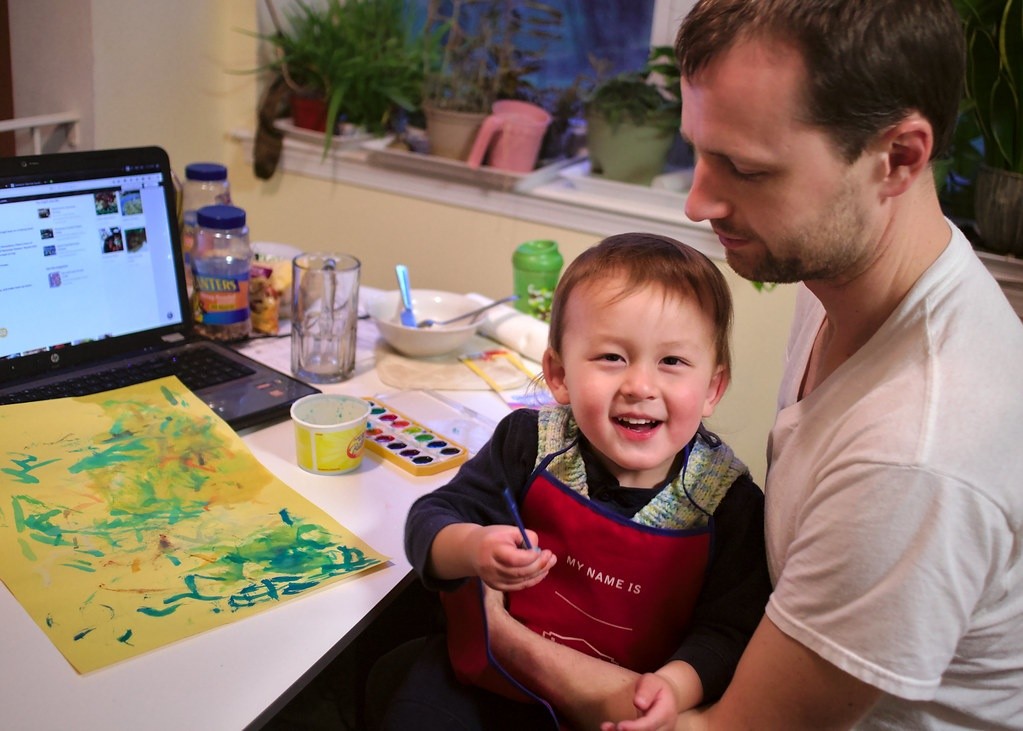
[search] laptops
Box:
[0,146,324,431]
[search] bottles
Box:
[188,205,252,342]
[181,164,233,291]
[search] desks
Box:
[0,282,543,731]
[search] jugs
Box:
[467,99,554,174]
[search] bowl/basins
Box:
[366,289,487,357]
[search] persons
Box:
[478,0,1023,731]
[395,231,771,731]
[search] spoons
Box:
[418,294,521,328]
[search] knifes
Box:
[396,264,417,327]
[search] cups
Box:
[290,392,372,473]
[289,251,362,384]
[511,239,563,323]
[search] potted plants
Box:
[217,0,1023,259]
